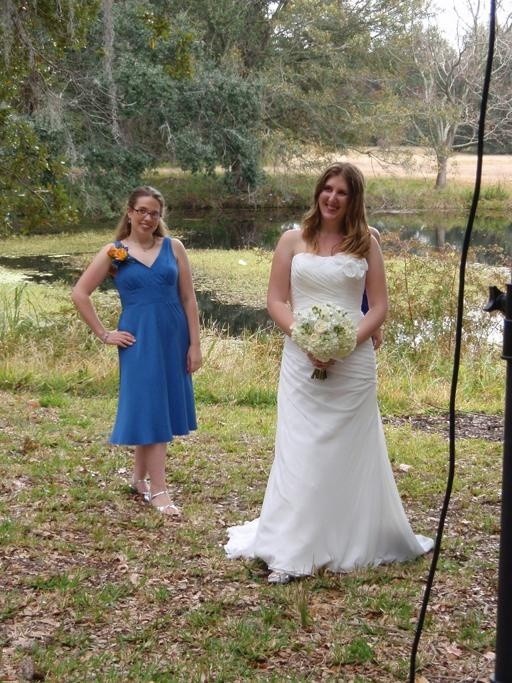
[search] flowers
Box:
[106,244,130,264]
[289,302,358,380]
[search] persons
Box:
[361,226,386,351]
[71,185,203,522]
[224,160,435,585]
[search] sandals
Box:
[149,490,181,520]
[129,473,150,502]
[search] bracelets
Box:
[102,331,110,345]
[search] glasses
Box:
[131,205,162,220]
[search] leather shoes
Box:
[268,571,292,584]
